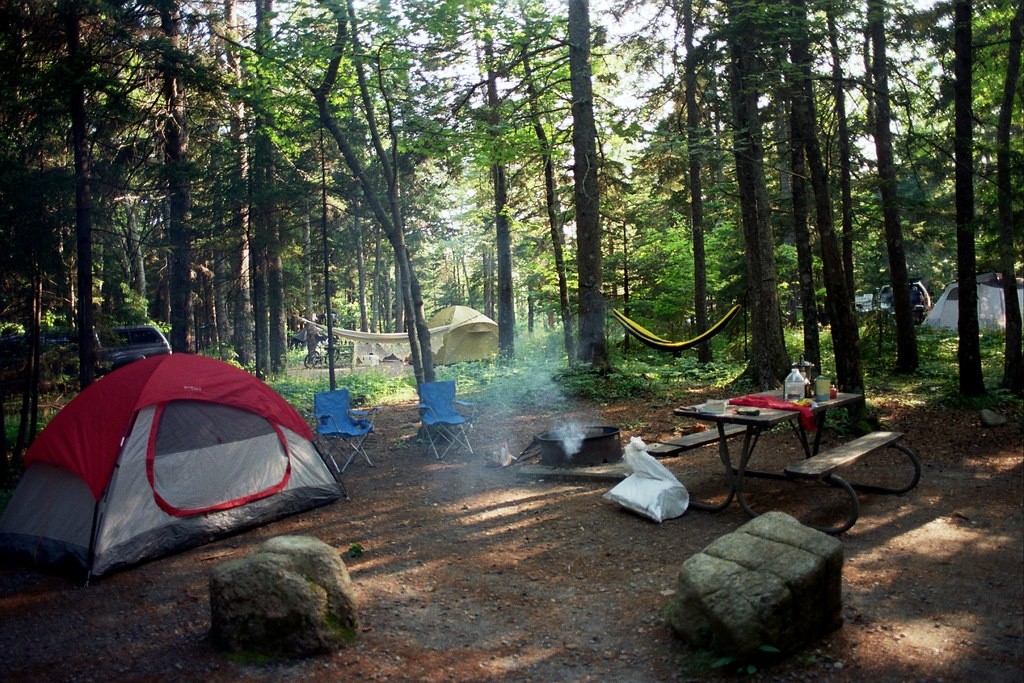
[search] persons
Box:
[785,295,798,328]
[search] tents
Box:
[920,272,1024,332]
[405,304,500,366]
[0,353,345,580]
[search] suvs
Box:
[877,280,932,316]
[0,324,172,392]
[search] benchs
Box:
[783,432,921,535]
[645,425,757,513]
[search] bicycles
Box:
[303,346,340,368]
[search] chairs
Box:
[416,380,476,460]
[311,388,384,475]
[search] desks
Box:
[672,387,863,516]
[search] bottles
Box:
[784,369,805,403]
